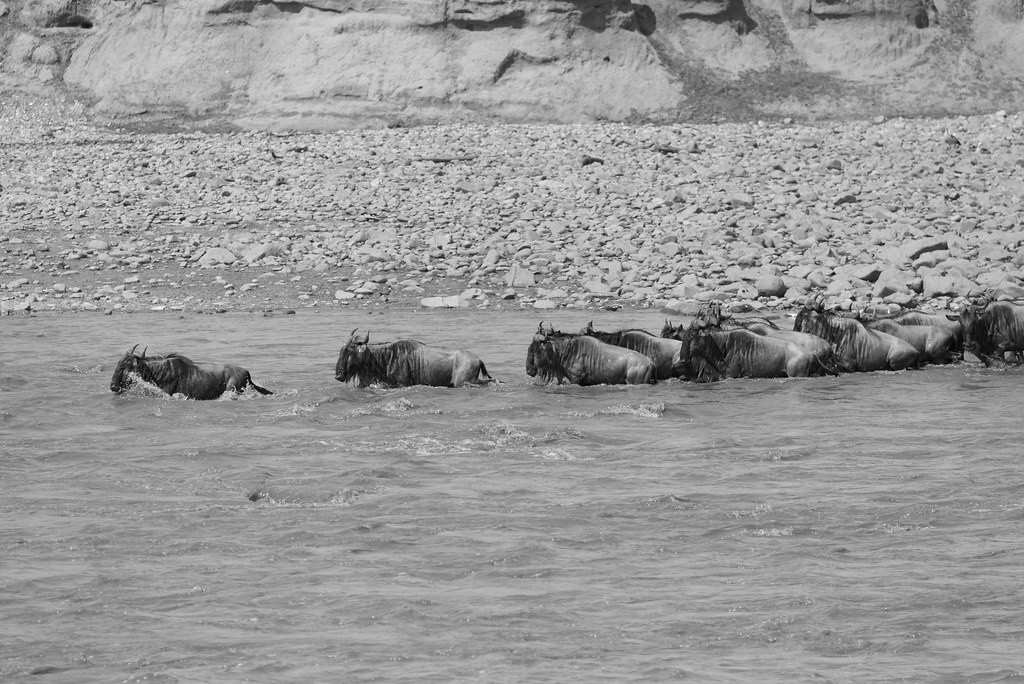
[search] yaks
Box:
[111,344,274,400]
[335,327,493,388]
[526,289,1024,386]
